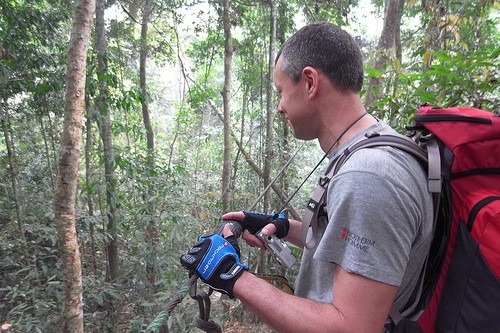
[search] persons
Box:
[179,21,436,333]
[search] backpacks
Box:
[300,102,499,333]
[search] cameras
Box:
[255,228,297,270]
